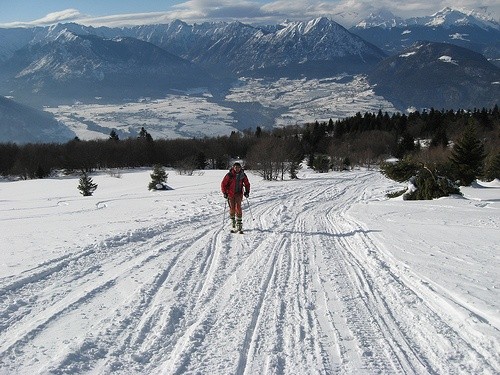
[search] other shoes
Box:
[237,230,243,234]
[231,228,236,232]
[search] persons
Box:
[220,162,250,234]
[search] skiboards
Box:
[230,228,244,234]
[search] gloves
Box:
[244,192,249,198]
[224,193,228,198]
